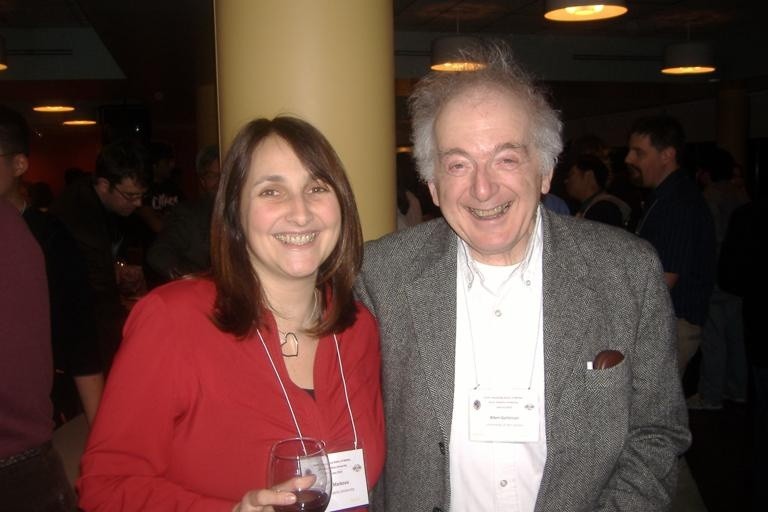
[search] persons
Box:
[337,36,706,512]
[72,114,387,512]
[0,97,767,468]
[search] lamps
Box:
[430,11,488,73]
[0,36,13,72]
[31,99,97,128]
[541,0,628,24]
[661,14,717,76]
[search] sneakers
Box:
[722,393,748,405]
[684,391,724,412]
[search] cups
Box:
[266,437,333,512]
[116,256,143,299]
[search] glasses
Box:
[113,185,150,200]
[202,171,220,179]
